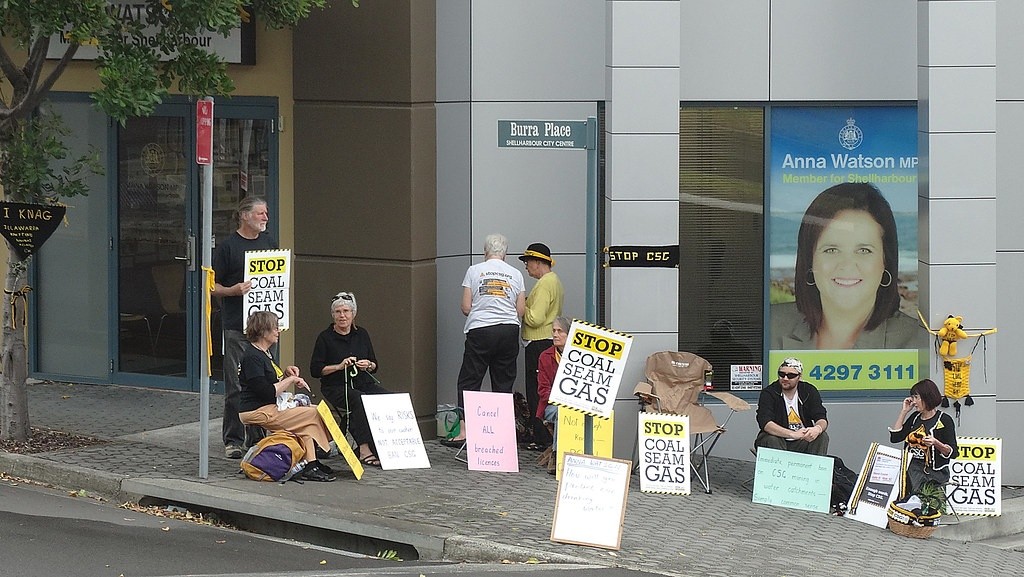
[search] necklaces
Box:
[254,343,272,359]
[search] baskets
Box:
[889,492,942,539]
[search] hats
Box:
[779,357,804,375]
[518,243,556,267]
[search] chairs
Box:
[151,262,219,350]
[630,351,751,499]
[119,312,153,354]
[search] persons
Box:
[440,233,527,450]
[310,292,391,466]
[236,310,338,482]
[696,320,753,389]
[519,241,564,452]
[534,316,573,474]
[754,357,830,455]
[767,180,920,351]
[210,196,281,458]
[888,379,960,494]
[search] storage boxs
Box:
[888,491,943,539]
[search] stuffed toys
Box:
[937,314,968,357]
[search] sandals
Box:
[441,437,467,450]
[522,441,547,451]
[302,463,336,482]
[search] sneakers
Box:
[225,439,258,458]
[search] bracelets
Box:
[369,362,372,369]
[816,425,822,433]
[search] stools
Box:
[536,418,557,475]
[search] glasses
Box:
[331,295,352,303]
[522,257,536,265]
[778,370,800,380]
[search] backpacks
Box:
[241,429,306,483]
